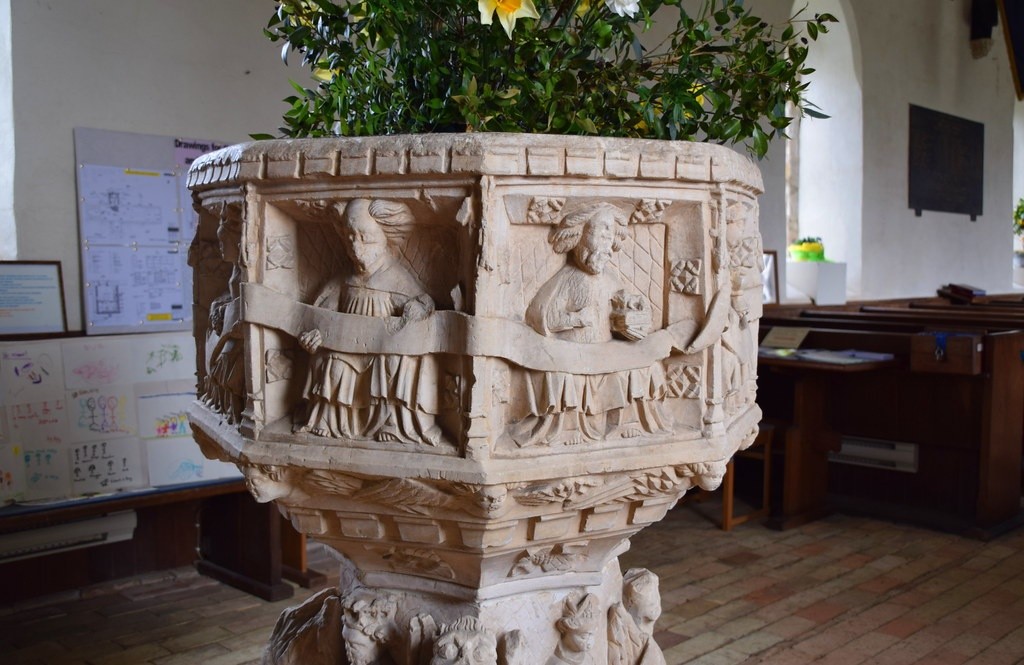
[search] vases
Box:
[1013,251,1024,268]
[183,134,763,665]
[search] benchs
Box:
[758,295,1024,541]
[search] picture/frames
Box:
[761,249,780,306]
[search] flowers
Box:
[246,0,848,165]
[1012,196,1024,254]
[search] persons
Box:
[511,204,669,447]
[339,567,667,665]
[296,200,445,446]
[202,200,244,424]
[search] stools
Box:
[679,421,776,532]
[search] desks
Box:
[0,472,297,603]
